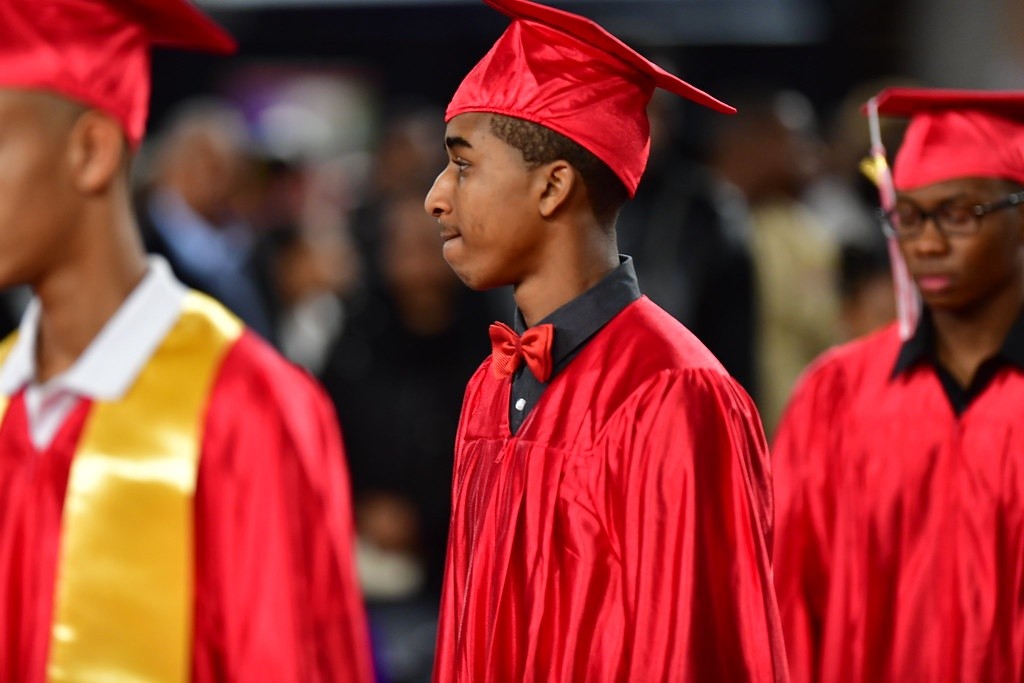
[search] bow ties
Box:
[488,320,555,383]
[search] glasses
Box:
[876,197,1020,235]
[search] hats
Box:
[854,88,1022,193]
[440,2,742,200]
[0,4,238,156]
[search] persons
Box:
[423,0,791,683]
[315,195,513,682]
[619,68,762,403]
[768,86,1024,683]
[138,107,363,376]
[751,99,898,437]
[0,0,377,683]
[834,232,901,336]
